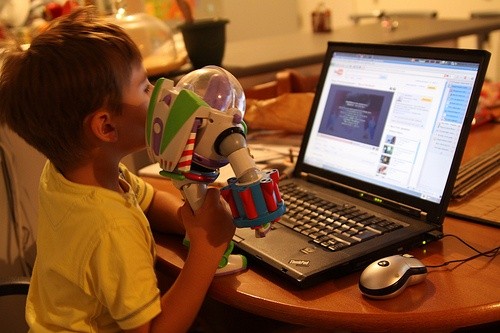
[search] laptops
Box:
[229,41,492,290]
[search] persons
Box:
[0,5,237,333]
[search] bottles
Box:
[312,1,332,33]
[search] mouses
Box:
[357,254,428,301]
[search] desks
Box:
[139,120,500,333]
[221,19,500,78]
